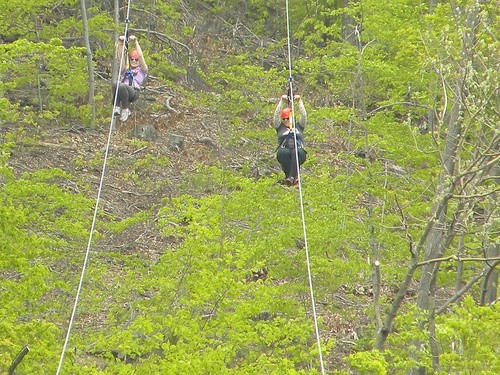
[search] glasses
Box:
[281,118,290,121]
[129,58,139,62]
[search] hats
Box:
[281,107,292,119]
[129,50,139,59]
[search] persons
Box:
[274,94,307,185]
[111,35,148,122]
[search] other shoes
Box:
[285,177,299,187]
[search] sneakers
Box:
[112,106,122,116]
[119,107,132,122]
